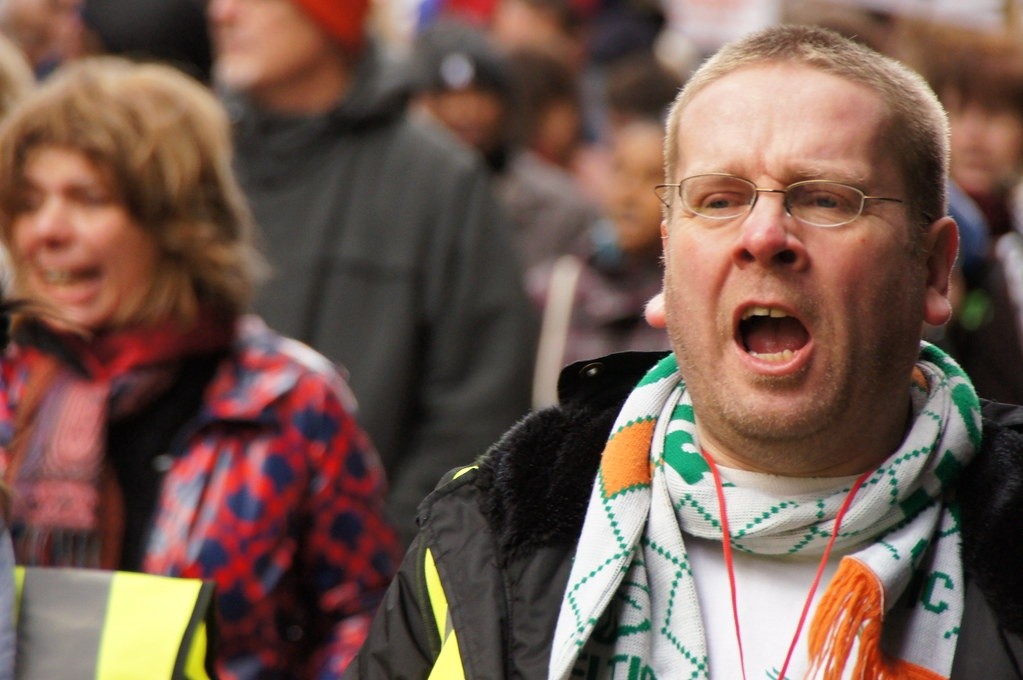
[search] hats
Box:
[295,0,370,55]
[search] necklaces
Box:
[702,449,876,680]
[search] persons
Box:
[343,26,1023,680]
[1,58,396,680]
[1,1,775,547]
[793,10,1022,408]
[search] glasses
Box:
[653,173,939,228]
